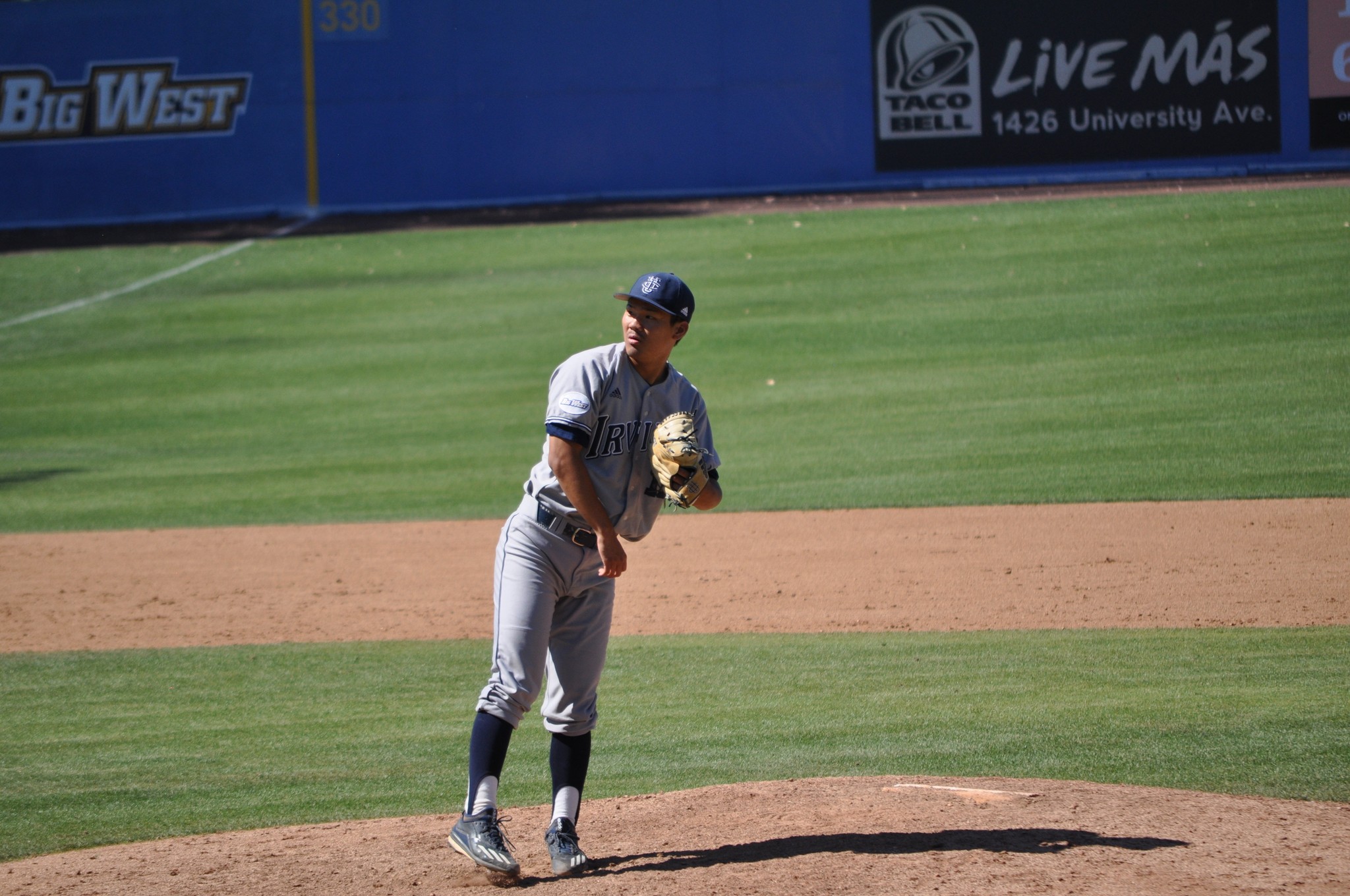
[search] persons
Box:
[447,271,724,881]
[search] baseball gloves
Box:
[651,411,710,510]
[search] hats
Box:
[613,273,696,323]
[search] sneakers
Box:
[447,806,520,876]
[545,816,588,877]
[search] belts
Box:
[537,508,598,549]
